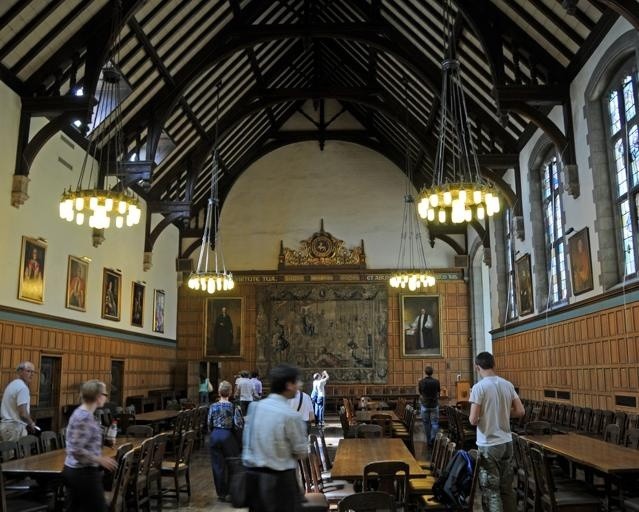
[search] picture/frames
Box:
[18,235,48,304]
[65,255,89,313]
[202,296,246,361]
[398,292,445,360]
[514,253,535,317]
[101,266,122,321]
[568,227,594,296]
[130,281,147,327]
[152,288,165,333]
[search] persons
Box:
[572,239,591,291]
[69,266,85,309]
[288,380,317,492]
[105,281,117,317]
[0,361,36,485]
[213,306,233,355]
[24,248,42,281]
[198,372,210,406]
[236,370,262,416]
[468,352,526,512]
[241,363,310,512]
[416,366,441,449]
[409,308,435,350]
[233,371,242,399]
[311,370,330,429]
[61,378,119,512]
[250,370,262,401]
[207,380,245,503]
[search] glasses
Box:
[101,392,110,397]
[26,368,37,374]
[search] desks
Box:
[357,411,399,438]
[521,433,639,511]
[0,436,149,510]
[132,409,179,458]
[328,438,425,510]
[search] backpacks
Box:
[427,449,474,512]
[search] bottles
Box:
[106,420,117,444]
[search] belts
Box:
[245,466,276,474]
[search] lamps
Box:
[187,80,235,293]
[389,72,436,292]
[58,0,142,229]
[415,1,504,227]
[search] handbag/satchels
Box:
[207,378,213,392]
[311,385,318,403]
[232,404,242,444]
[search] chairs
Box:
[407,436,450,484]
[510,397,525,424]
[318,429,359,484]
[419,449,481,512]
[308,434,349,486]
[149,430,196,502]
[125,425,153,439]
[589,409,603,436]
[407,444,455,512]
[576,408,592,434]
[603,431,638,511]
[531,446,602,512]
[525,421,558,480]
[0,441,48,512]
[124,436,156,511]
[138,433,167,512]
[517,437,570,512]
[183,409,193,437]
[362,460,404,512]
[511,399,533,431]
[40,431,68,511]
[58,426,69,448]
[96,404,137,425]
[112,414,136,436]
[161,410,184,461]
[553,405,574,431]
[611,412,627,445]
[512,433,532,511]
[102,449,135,512]
[446,406,478,453]
[599,409,614,440]
[591,424,620,495]
[301,451,357,512]
[522,401,543,433]
[394,403,417,447]
[308,442,354,494]
[17,435,70,511]
[567,408,582,432]
[337,490,396,512]
[192,407,208,452]
[542,402,556,425]
[104,443,135,511]
[411,432,443,478]
[625,415,639,448]
[339,398,364,439]
